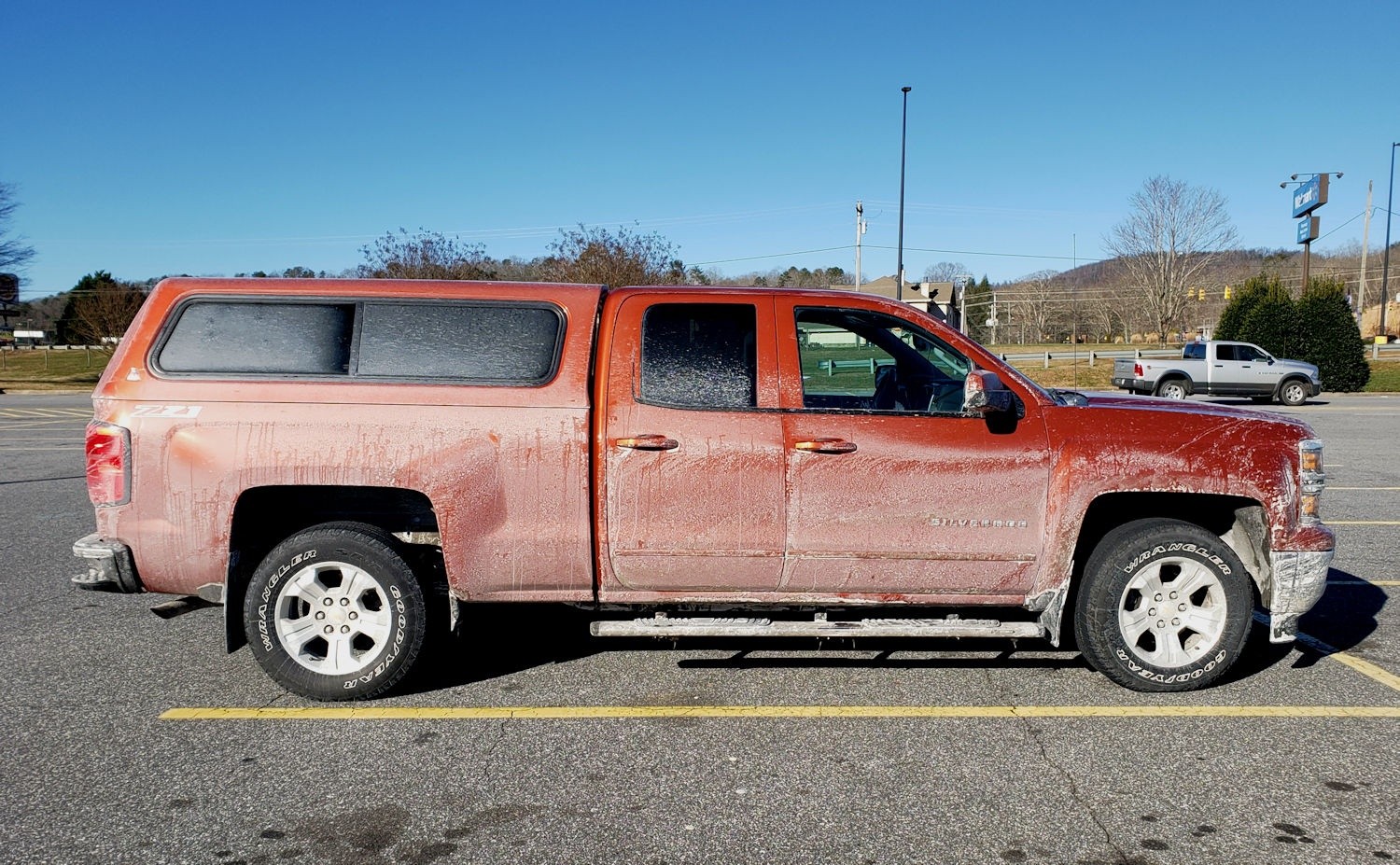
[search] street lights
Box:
[897,86,912,303]
[1279,171,1345,299]
[1374,141,1400,345]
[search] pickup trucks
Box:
[1110,341,1322,406]
[71,277,1338,704]
